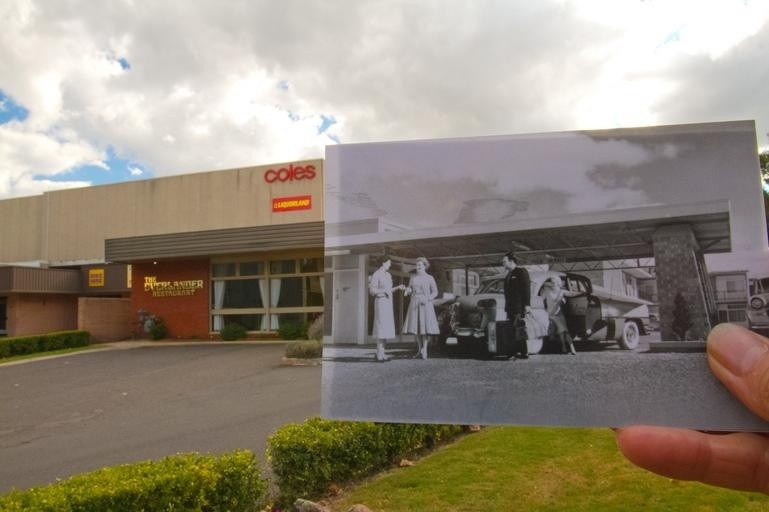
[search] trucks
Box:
[438,270,651,358]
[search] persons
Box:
[617,322,769,492]
[501,252,532,360]
[538,276,586,354]
[368,256,407,363]
[401,257,441,360]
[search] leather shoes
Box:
[375,355,391,363]
[509,350,529,360]
